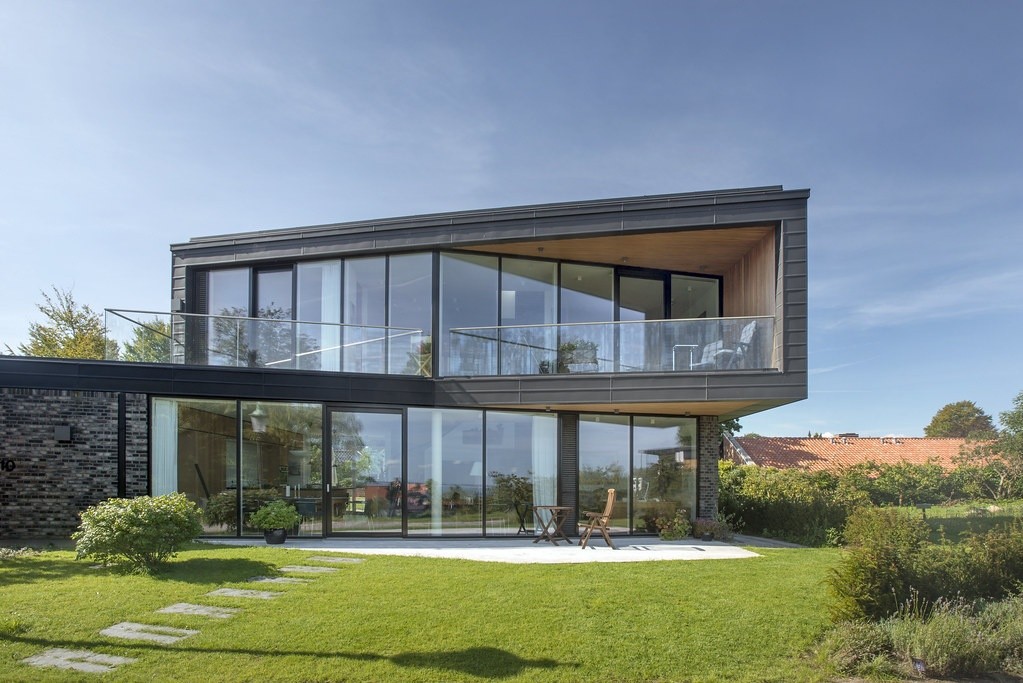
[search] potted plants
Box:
[700,518,712,541]
[249,499,303,546]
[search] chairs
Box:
[712,319,757,369]
[689,340,724,370]
[296,500,317,518]
[576,487,618,549]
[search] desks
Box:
[673,344,699,371]
[512,502,529,538]
[531,505,573,546]
[302,497,347,508]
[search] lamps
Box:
[247,403,268,433]
[470,461,488,533]
